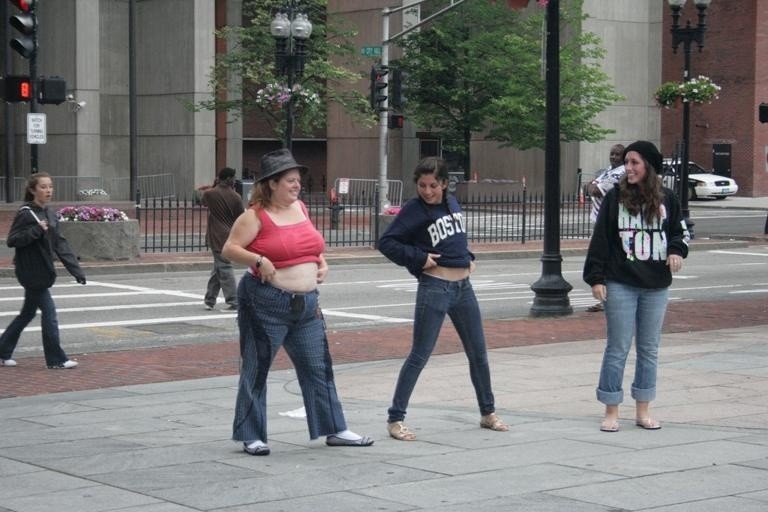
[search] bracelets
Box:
[256,254,264,269]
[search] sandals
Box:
[387,421,417,441]
[480,413,510,431]
[599,419,619,432]
[636,417,661,430]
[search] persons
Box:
[198,168,245,310]
[583,141,690,431]
[1,172,86,370]
[220,148,374,455]
[378,158,509,441]
[586,144,626,312]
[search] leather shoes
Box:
[243,441,269,456]
[326,435,374,446]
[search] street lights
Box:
[668,1,711,240]
[271,12,313,152]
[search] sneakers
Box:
[48,360,78,369]
[0,358,16,366]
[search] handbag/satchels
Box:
[15,238,56,289]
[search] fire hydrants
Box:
[327,199,344,229]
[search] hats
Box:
[623,141,662,167]
[253,148,310,183]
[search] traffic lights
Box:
[4,75,31,104]
[394,70,408,111]
[388,116,403,131]
[9,0,37,58]
[370,67,389,119]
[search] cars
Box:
[193,185,213,207]
[658,157,738,201]
